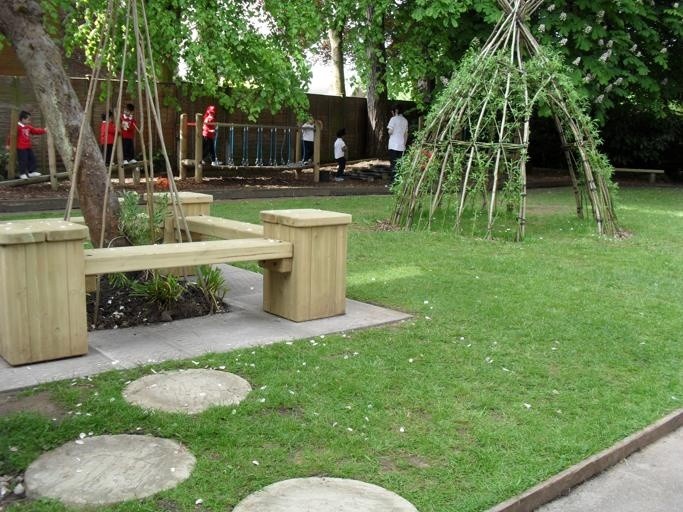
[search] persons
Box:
[302,115,324,164]
[100,109,121,166]
[120,104,136,164]
[334,128,348,182]
[6,111,47,179]
[201,106,221,167]
[385,103,409,187]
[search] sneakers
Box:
[211,161,222,165]
[19,171,41,179]
[124,159,138,164]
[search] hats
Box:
[206,105,215,113]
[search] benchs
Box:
[607,168,664,184]
